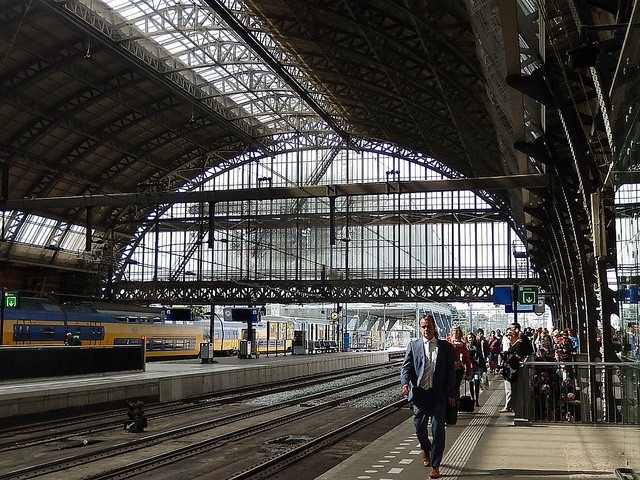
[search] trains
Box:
[2,298,333,361]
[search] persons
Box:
[487,329,502,376]
[552,326,578,423]
[449,324,471,404]
[401,314,456,479]
[476,329,489,389]
[462,333,483,407]
[499,320,552,413]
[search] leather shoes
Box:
[423,453,430,467]
[430,466,439,477]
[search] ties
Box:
[419,341,431,390]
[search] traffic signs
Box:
[517,285,540,306]
[4,291,19,309]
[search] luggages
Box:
[459,376,474,412]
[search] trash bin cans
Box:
[199,342,213,363]
[240,340,252,359]
